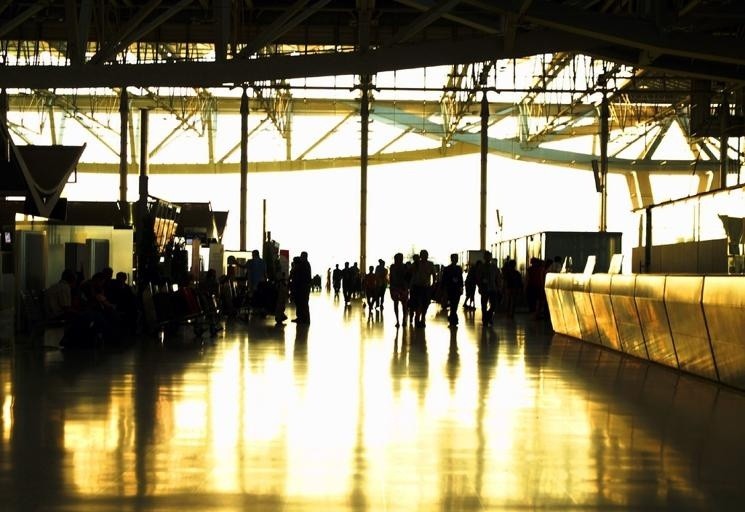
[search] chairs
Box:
[19,279,252,352]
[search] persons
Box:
[44,250,322,366]
[326,244,561,329]
